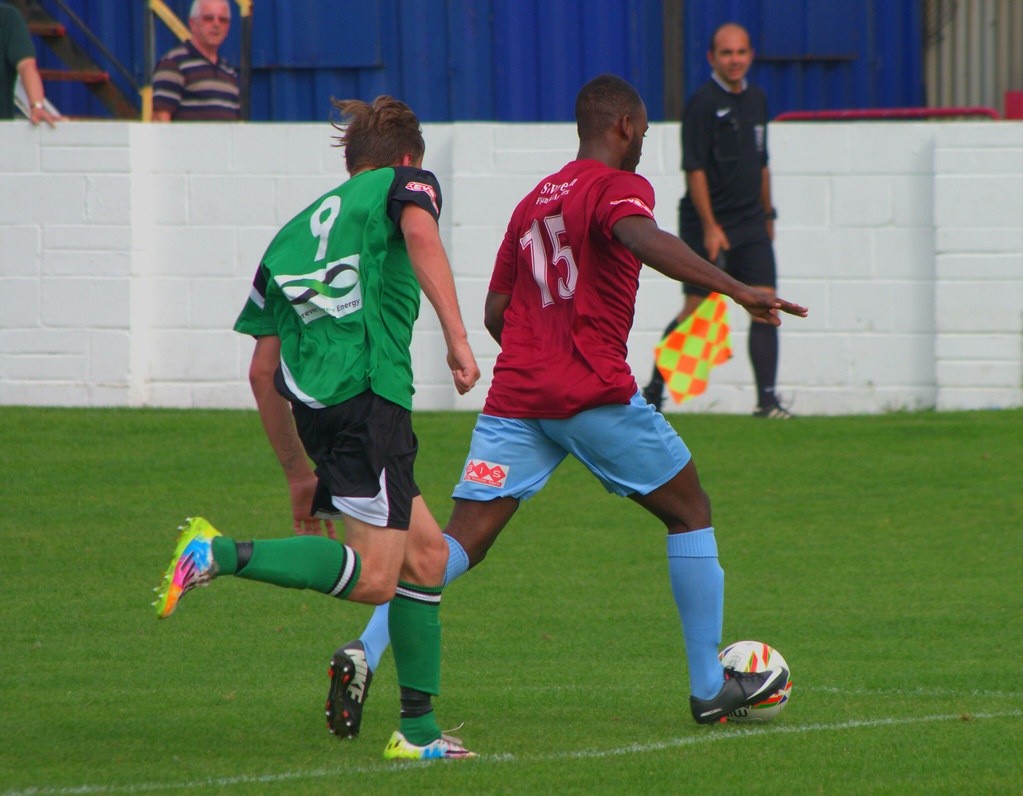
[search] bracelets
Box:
[765,209,777,221]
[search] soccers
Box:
[718,640,793,723]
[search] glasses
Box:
[190,14,232,26]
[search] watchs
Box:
[31,103,43,108]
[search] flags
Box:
[654,291,734,404]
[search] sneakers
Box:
[687,663,790,728]
[381,727,482,763]
[324,640,378,740]
[642,381,668,414]
[749,401,800,420]
[153,514,226,621]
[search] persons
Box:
[151,0,240,123]
[324,74,808,740]
[0,2,69,128]
[151,94,480,760]
[641,22,794,418]
